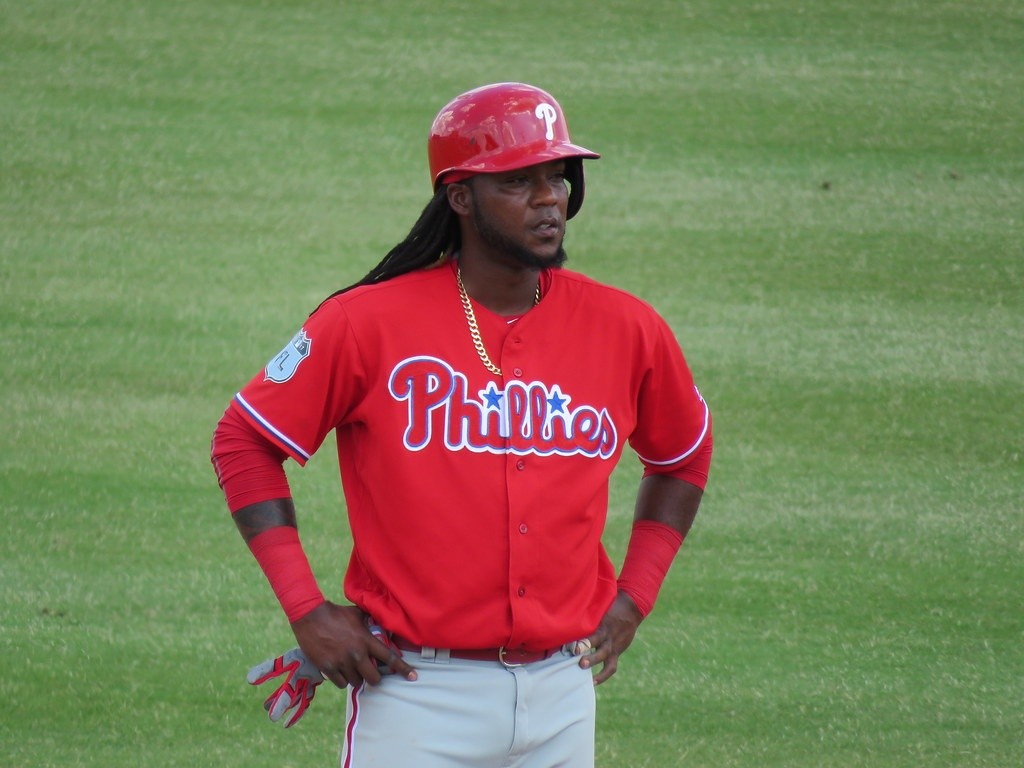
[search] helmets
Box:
[429,82,601,183]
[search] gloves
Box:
[247,615,402,727]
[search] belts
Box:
[388,618,563,668]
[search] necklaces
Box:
[455,267,541,378]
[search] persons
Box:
[209,82,714,768]
